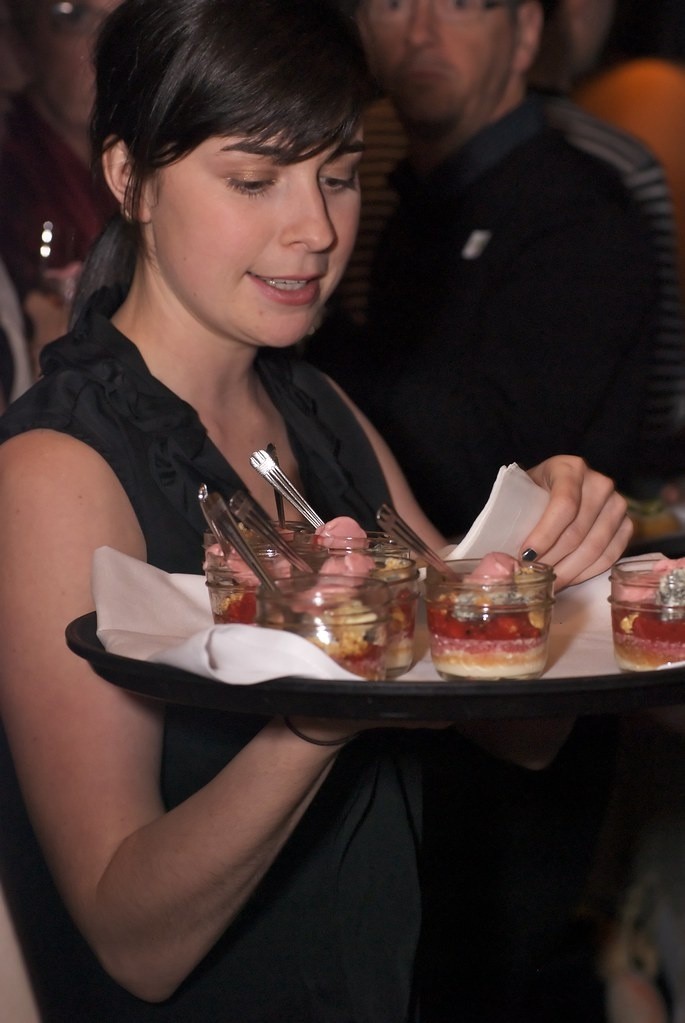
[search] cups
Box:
[607,558,685,672]
[202,518,420,681]
[32,218,78,293]
[419,558,558,681]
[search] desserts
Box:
[206,515,685,680]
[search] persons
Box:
[0,0,685,1023]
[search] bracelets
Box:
[283,711,356,747]
